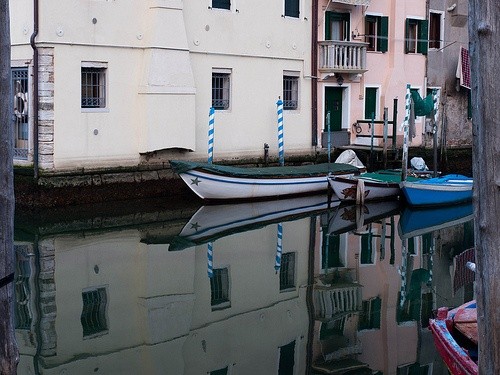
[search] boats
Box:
[167,189,341,251]
[429,298,478,375]
[396,205,474,241]
[326,168,440,202]
[400,173,474,206]
[168,160,361,199]
[326,196,403,235]
